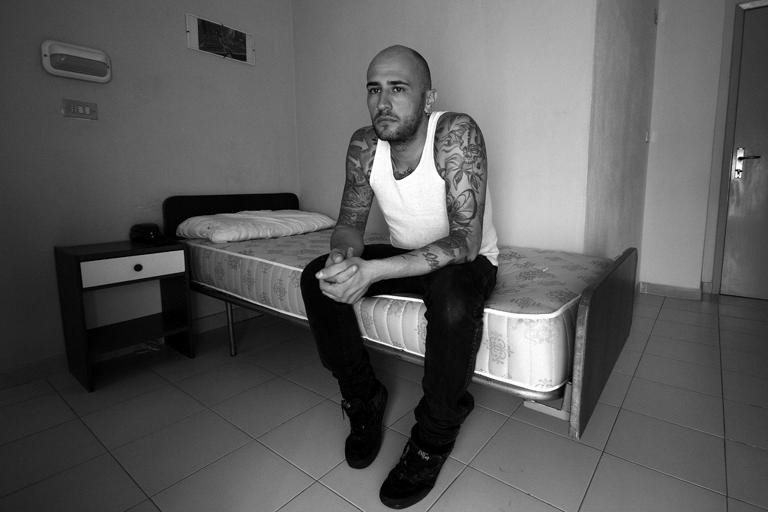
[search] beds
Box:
[162,193,638,441]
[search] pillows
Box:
[175,210,338,244]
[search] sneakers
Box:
[341,381,391,470]
[379,439,455,510]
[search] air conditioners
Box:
[41,39,111,83]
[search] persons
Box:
[300,45,500,510]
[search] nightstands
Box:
[54,241,197,393]
